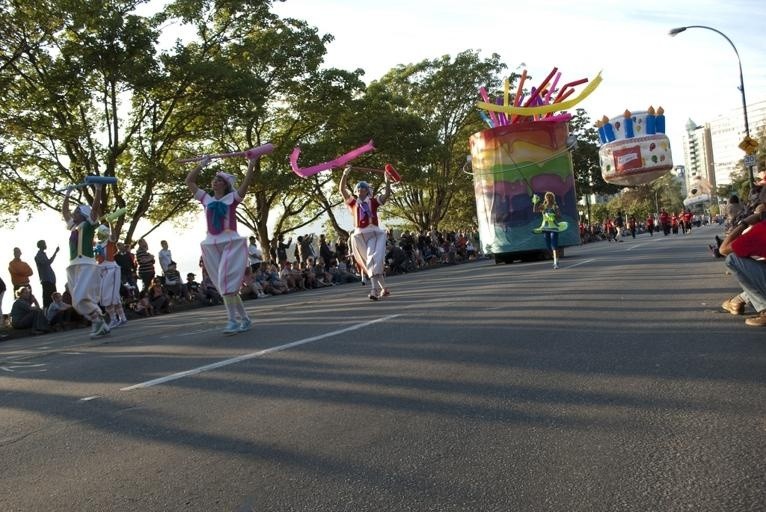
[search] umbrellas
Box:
[745,312,766,326]
[721,296,744,315]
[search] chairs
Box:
[89,317,128,339]
[222,317,254,337]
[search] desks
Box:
[670,24,756,204]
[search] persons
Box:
[92,219,128,330]
[0,222,477,337]
[530,190,562,270]
[184,151,261,336]
[338,165,391,302]
[716,163,766,327]
[62,183,110,340]
[580,207,724,242]
